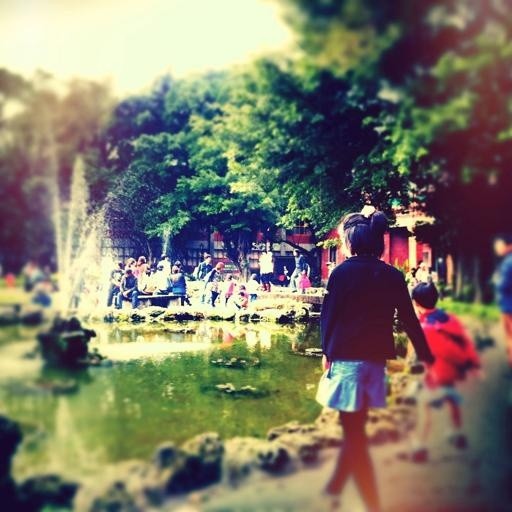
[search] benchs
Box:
[113,293,187,308]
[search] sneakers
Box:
[447,436,466,448]
[401,448,427,463]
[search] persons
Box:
[313,213,482,510]
[108,248,310,307]
[23,258,59,293]
[489,236,512,367]
